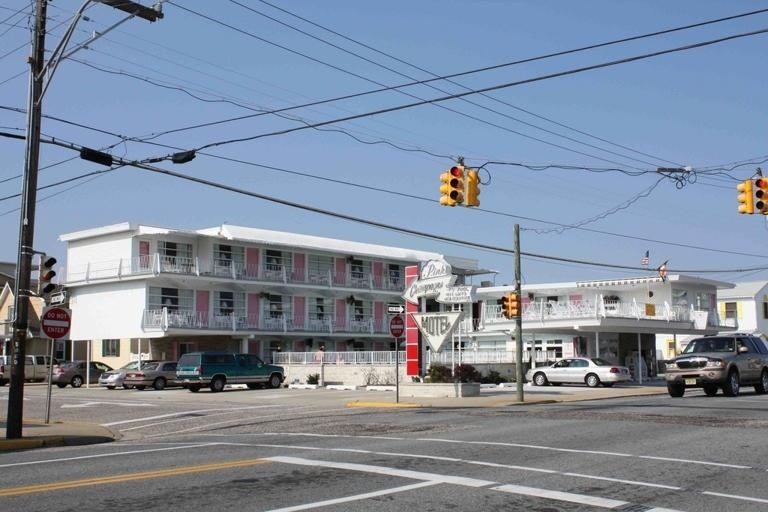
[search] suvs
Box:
[664,332,768,398]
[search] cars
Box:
[123,360,186,390]
[525,357,631,387]
[49,360,113,388]
[98,361,141,389]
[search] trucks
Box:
[0,354,58,383]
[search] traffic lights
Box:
[510,293,519,316]
[502,293,511,317]
[35,252,57,296]
[466,169,483,206]
[736,180,755,214]
[755,178,768,215]
[440,166,464,207]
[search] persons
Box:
[314,345,326,366]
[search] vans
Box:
[176,350,284,393]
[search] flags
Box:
[656,260,670,283]
[640,250,648,269]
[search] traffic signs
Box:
[389,316,406,338]
[39,306,73,340]
